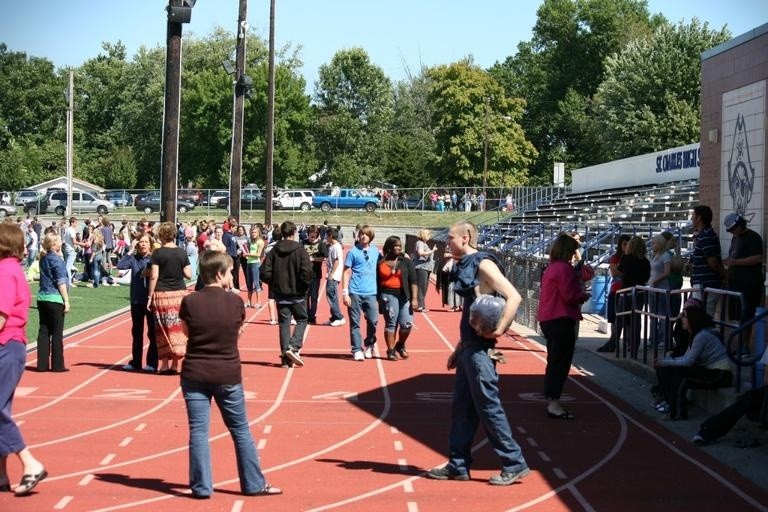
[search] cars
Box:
[1,205,17,218]
[24,196,47,217]
[110,192,133,207]
[15,191,39,206]
[134,190,318,215]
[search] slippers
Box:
[14,470,48,495]
[546,406,573,420]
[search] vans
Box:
[46,190,114,215]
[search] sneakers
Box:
[231,287,240,294]
[354,343,409,361]
[102,283,120,286]
[692,433,706,444]
[428,467,471,480]
[650,393,672,421]
[489,467,529,485]
[281,347,304,367]
[269,319,297,325]
[306,317,345,327]
[244,304,262,308]
[123,363,177,374]
[415,306,429,312]
[447,306,460,312]
[597,344,615,353]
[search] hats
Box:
[724,214,739,229]
[185,226,194,241]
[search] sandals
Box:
[250,484,282,495]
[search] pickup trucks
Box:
[311,190,378,213]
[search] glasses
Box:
[364,250,369,260]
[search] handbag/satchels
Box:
[409,252,430,266]
[581,264,595,281]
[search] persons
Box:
[599,206,735,420]
[721,213,764,358]
[539,231,597,419]
[16,212,514,376]
[367,190,408,211]
[428,190,512,211]
[178,251,282,499]
[0,221,47,496]
[426,219,530,486]
[692,345,768,445]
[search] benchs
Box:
[481,180,697,287]
